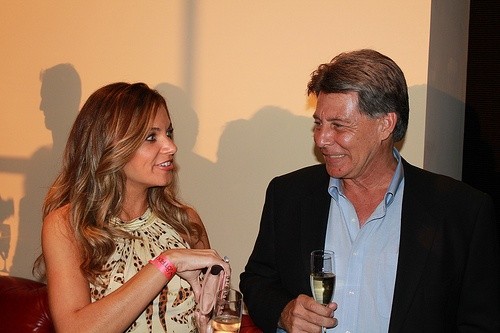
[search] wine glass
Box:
[310,250,336,333]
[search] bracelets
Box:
[149,253,177,282]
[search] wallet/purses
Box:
[199,266,226,315]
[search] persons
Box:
[238,48,499,333]
[29,82,232,333]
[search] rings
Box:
[221,255,230,265]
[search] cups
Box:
[211,288,243,333]
